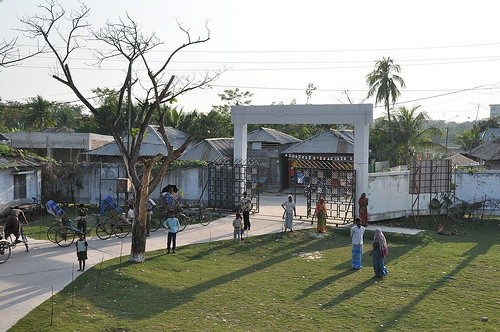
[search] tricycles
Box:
[160,191,212,232]
[0,223,29,265]
[100,195,162,238]
[44,200,114,248]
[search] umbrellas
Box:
[146,197,156,211]
[161,184,179,194]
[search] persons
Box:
[357,192,369,228]
[73,203,89,241]
[437,223,446,234]
[126,204,135,226]
[169,187,177,201]
[280,194,297,234]
[314,197,328,234]
[232,212,245,243]
[239,191,253,230]
[3,205,28,244]
[369,228,389,278]
[349,216,366,270]
[75,232,89,273]
[146,210,153,237]
[451,224,459,236]
[163,212,180,254]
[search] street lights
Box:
[442,113,460,153]
